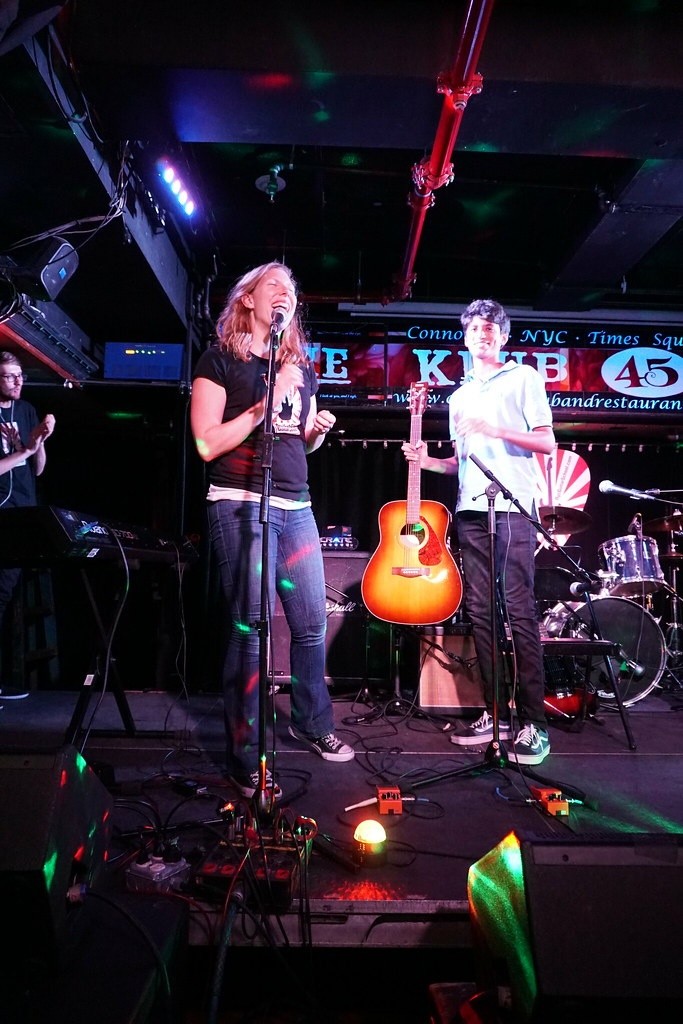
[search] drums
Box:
[597,535,665,599]
[543,594,668,709]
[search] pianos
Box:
[0,504,205,753]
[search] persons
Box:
[0,351,55,708]
[400,301,555,764]
[187,263,355,762]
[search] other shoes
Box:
[0,704,3,710]
[0,687,29,700]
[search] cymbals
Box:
[657,551,683,560]
[536,506,593,535]
[618,514,683,535]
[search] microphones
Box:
[599,480,656,501]
[268,308,288,334]
[626,659,646,677]
[462,661,473,671]
[628,514,639,532]
[569,581,601,596]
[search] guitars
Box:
[533,597,598,723]
[360,382,464,627]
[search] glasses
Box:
[0,374,27,382]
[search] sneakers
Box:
[507,723,550,765]
[450,711,515,745]
[228,768,282,799]
[288,725,355,762]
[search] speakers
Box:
[266,552,400,693]
[520,830,683,1024]
[413,625,488,716]
[0,744,114,969]
[19,236,80,303]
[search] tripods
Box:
[119,333,364,876]
[397,440,600,810]
[666,558,683,669]
